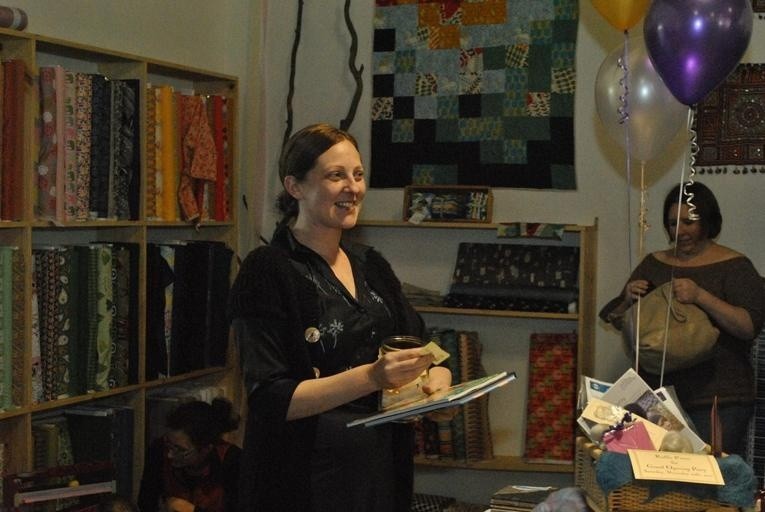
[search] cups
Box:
[377,335,429,424]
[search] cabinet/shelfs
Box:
[0,23,239,512]
[352,219,598,486]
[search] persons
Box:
[598,181,765,460]
[135,398,247,511]
[229,123,457,509]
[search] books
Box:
[489,483,562,512]
[346,370,510,428]
[576,367,728,487]
[365,371,518,430]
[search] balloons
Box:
[595,35,689,166]
[644,2,755,109]
[588,1,654,32]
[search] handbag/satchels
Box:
[620,280,721,384]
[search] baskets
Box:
[573,436,756,512]
